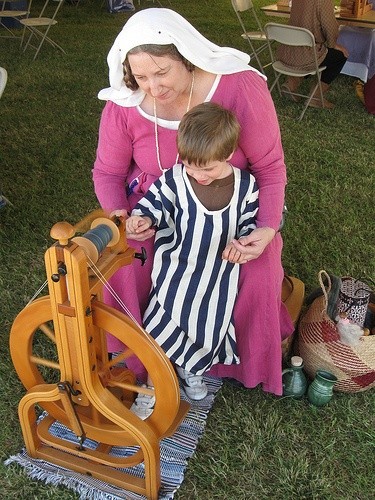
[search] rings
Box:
[246,259,250,263]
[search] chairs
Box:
[0,0,66,60]
[231,0,282,97]
[263,22,327,122]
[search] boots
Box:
[304,79,335,109]
[281,75,304,102]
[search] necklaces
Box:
[154,71,195,172]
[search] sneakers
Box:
[129,384,156,421]
[182,376,208,401]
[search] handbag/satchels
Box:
[296,269,375,393]
[109,0,136,14]
[280,270,305,362]
[336,276,375,327]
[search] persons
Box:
[125,102,260,420]
[92,9,295,396]
[277,0,350,109]
[353,73,375,114]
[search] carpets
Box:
[4,352,223,500]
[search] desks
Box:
[260,4,375,28]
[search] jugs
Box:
[307,371,338,407]
[281,356,307,398]
